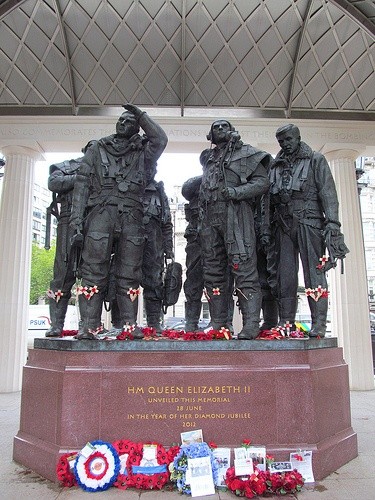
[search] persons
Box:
[45,103,350,340]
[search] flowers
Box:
[47,253,330,341]
[55,440,304,499]
